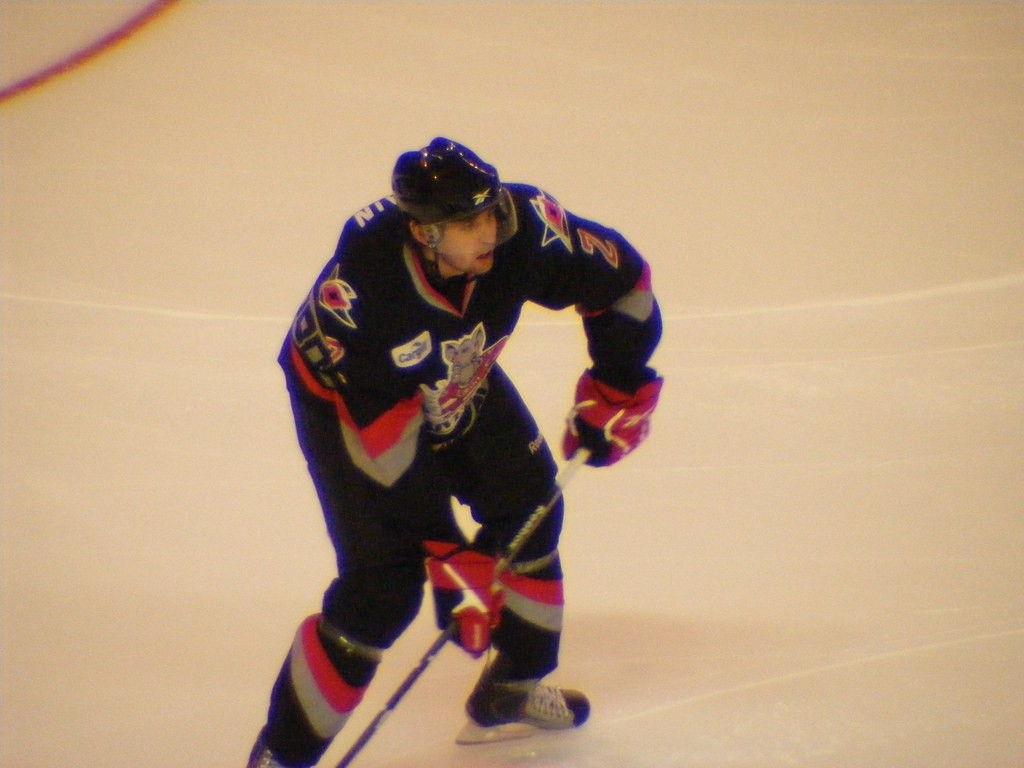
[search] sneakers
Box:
[245,723,282,768]
[456,665,589,744]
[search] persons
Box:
[245,134,664,768]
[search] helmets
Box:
[391,137,501,224]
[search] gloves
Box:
[563,367,663,467]
[425,551,504,657]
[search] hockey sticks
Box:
[332,444,592,767]
